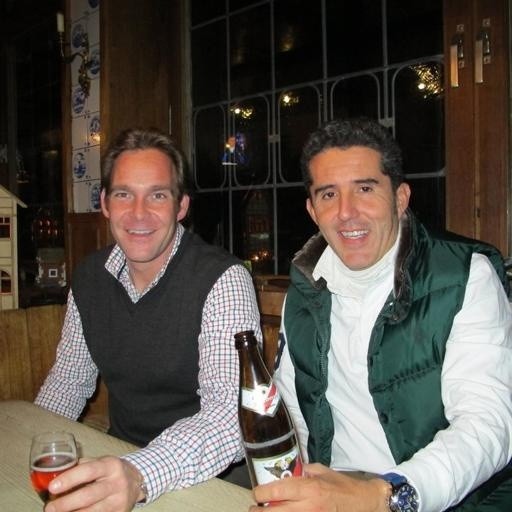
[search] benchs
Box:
[1,285,287,433]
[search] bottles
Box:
[234,330,306,508]
[30,208,59,248]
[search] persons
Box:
[33,128,264,512]
[248,116,512,512]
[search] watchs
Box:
[382,471,419,512]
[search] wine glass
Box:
[28,431,79,505]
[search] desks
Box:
[0,399,254,512]
